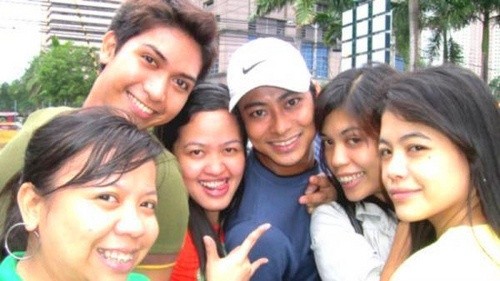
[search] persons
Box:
[310,64,417,281]
[0,104,172,281]
[226,38,342,281]
[0,0,216,281]
[170,82,273,281]
[379,64,500,281]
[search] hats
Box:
[224,36,311,114]
